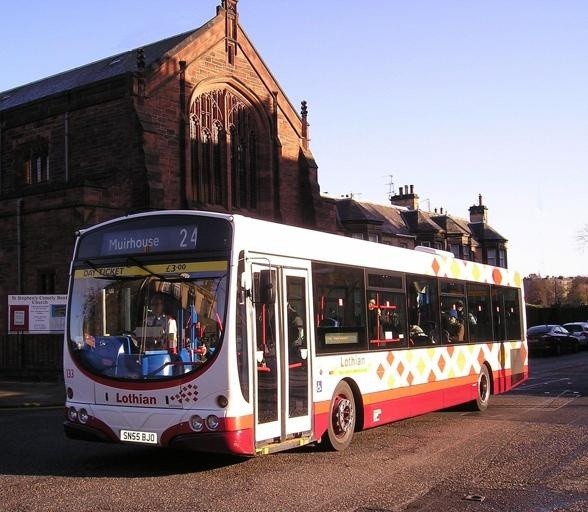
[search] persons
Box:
[358,297,478,346]
[142,293,178,357]
[272,297,303,349]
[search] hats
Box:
[449,309,458,318]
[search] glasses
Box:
[150,303,163,305]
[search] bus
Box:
[63,209,528,457]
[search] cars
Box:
[528,322,588,355]
